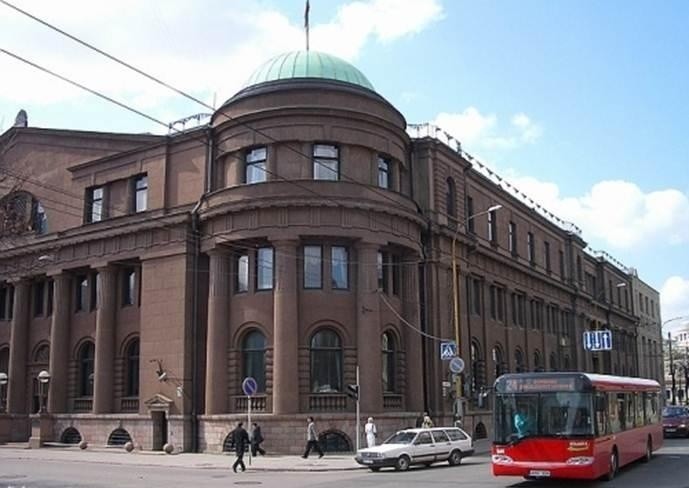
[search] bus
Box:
[490,371,664,480]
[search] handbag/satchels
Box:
[258,436,264,444]
[374,433,377,437]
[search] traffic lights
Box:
[346,382,359,399]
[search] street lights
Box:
[0,373,8,413]
[39,371,50,412]
[596,281,626,372]
[452,203,505,427]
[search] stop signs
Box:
[242,378,257,394]
[450,357,464,372]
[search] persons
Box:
[454,413,463,428]
[573,411,585,427]
[302,417,324,458]
[251,423,265,456]
[365,416,377,447]
[514,404,534,438]
[230,422,251,472]
[416,409,432,428]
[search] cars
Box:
[355,427,475,471]
[662,403,689,437]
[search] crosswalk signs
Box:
[441,343,456,361]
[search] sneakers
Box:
[319,454,323,460]
[302,456,306,458]
[232,466,236,472]
